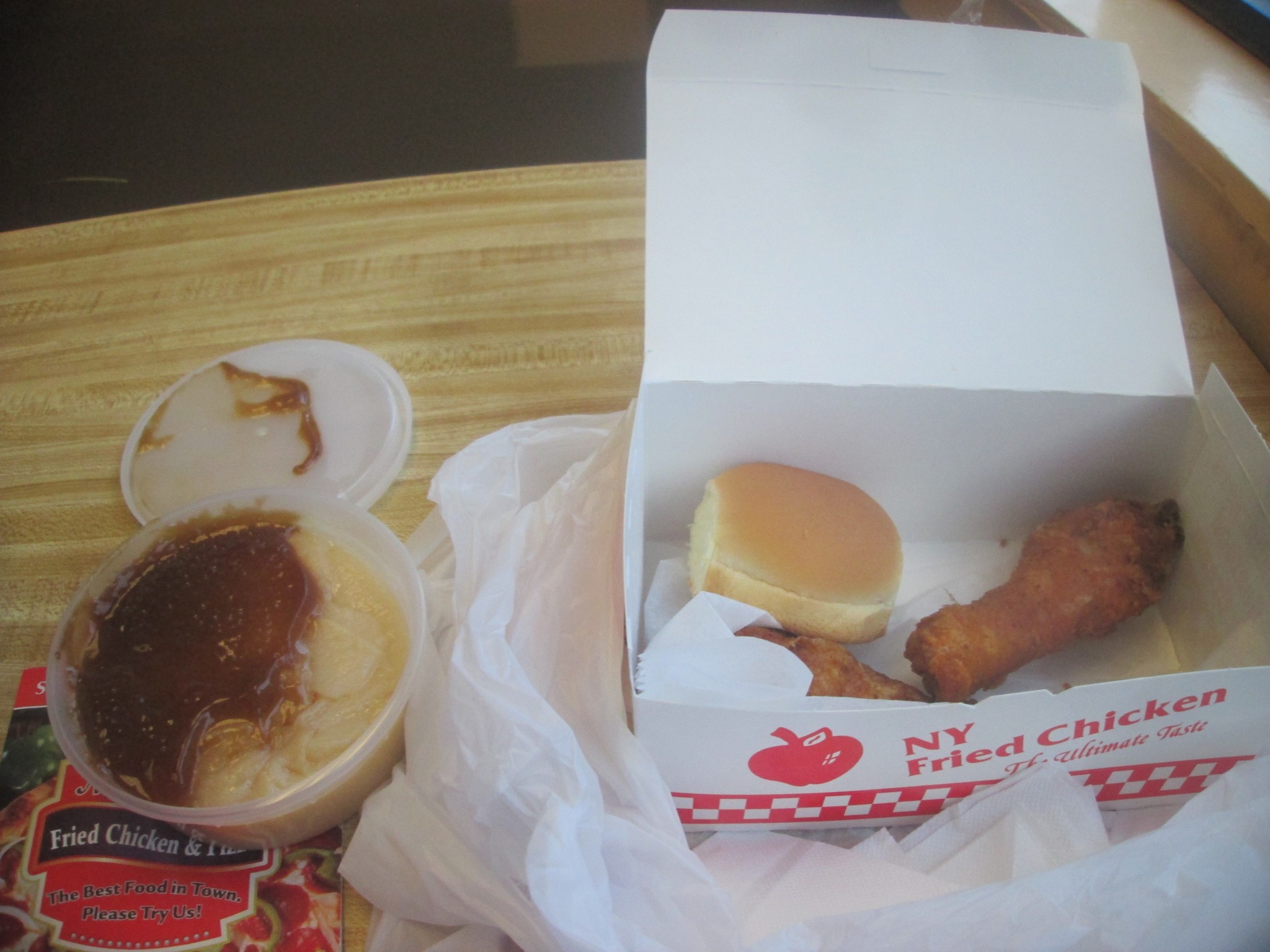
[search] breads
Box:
[688,462,901,645]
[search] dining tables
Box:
[0,155,1270,952]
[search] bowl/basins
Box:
[46,483,428,849]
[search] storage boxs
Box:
[622,7,1270,830]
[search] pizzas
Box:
[0,773,342,952]
